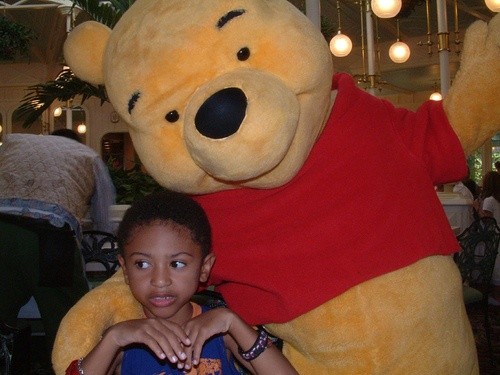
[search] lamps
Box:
[329,0,352,58]
[389,17,410,64]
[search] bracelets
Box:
[240,329,268,360]
[65,357,84,375]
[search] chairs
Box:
[80,230,122,280]
[453,216,500,367]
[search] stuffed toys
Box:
[51,0,500,375]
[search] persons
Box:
[66,192,300,375]
[0,128,116,375]
[452,161,500,305]
